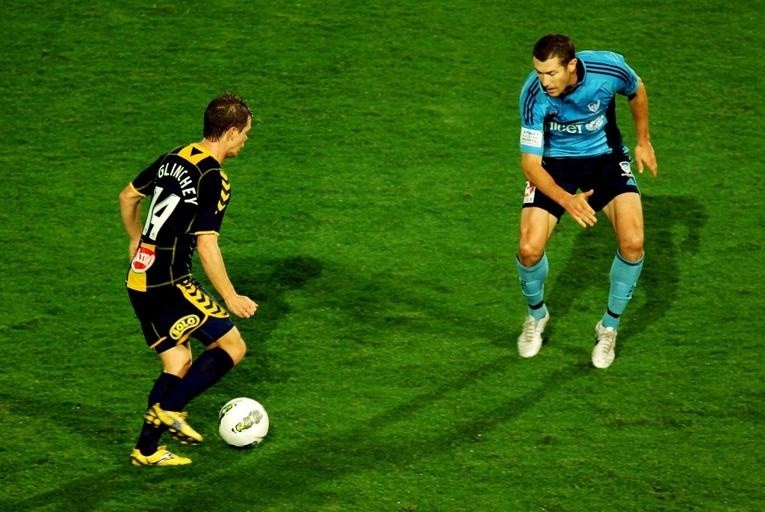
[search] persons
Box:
[117,93,259,467]
[516,34,659,370]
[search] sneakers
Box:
[142,402,204,447]
[517,308,549,359]
[131,445,193,468]
[591,320,618,369]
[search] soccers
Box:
[218,396,270,450]
